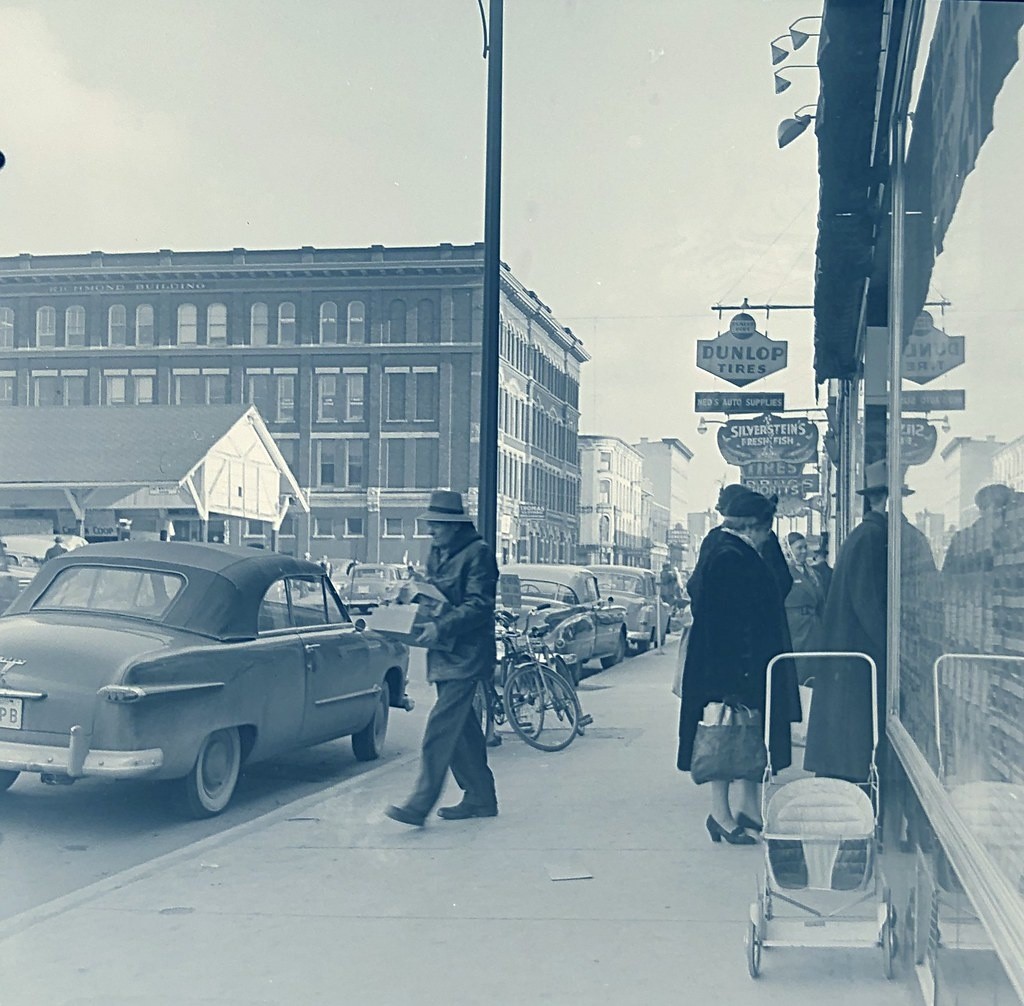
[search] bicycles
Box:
[472,604,593,752]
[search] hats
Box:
[416,490,475,521]
[725,492,768,517]
[855,458,888,495]
[54,536,63,543]
[304,552,311,558]
[715,484,752,510]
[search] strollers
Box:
[747,652,898,980]
[908,653,1024,960]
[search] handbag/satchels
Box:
[690,702,769,786]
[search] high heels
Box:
[736,811,762,832]
[706,814,757,845]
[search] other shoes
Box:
[383,805,424,826]
[436,801,498,820]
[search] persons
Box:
[45,537,67,561]
[383,490,498,827]
[677,484,802,845]
[347,557,362,577]
[304,553,333,578]
[804,460,888,791]
[407,565,416,575]
[900,486,1024,855]
[662,564,678,616]
[780,531,832,744]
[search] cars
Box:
[0,533,415,819]
[495,563,631,686]
[340,563,402,614]
[584,565,671,657]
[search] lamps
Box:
[771,12,889,148]
